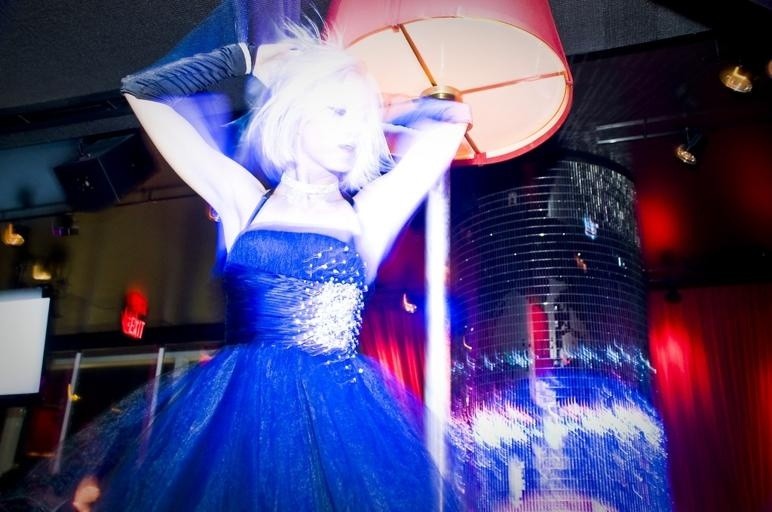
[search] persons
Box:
[40,14,474,511]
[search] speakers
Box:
[53,131,158,213]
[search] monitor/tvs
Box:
[0,282,58,406]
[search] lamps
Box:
[673,119,715,164]
[3,224,29,248]
[718,63,752,92]
[322,0,574,167]
